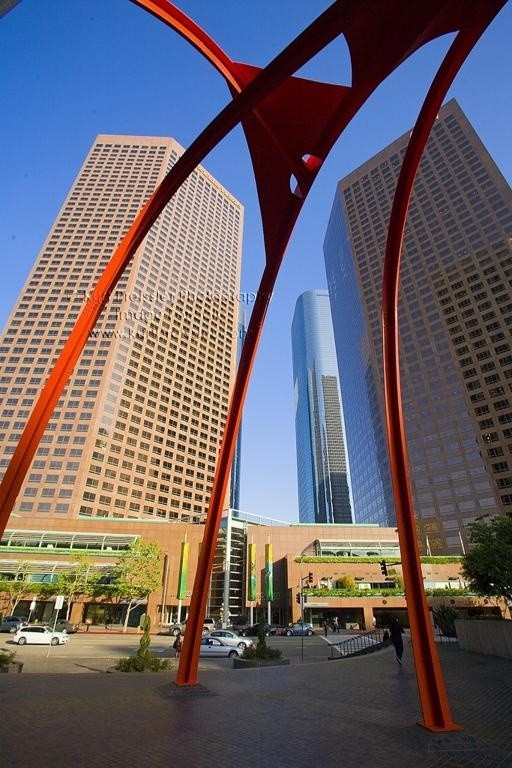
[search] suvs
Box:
[13,624,71,645]
[168,617,218,636]
[0,615,29,634]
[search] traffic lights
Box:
[304,595,308,604]
[309,572,314,583]
[379,560,388,576]
[296,593,300,604]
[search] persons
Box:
[372,615,376,629]
[332,621,340,632]
[389,614,405,665]
[323,623,327,637]
[174,633,183,658]
[382,628,391,644]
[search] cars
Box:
[198,635,243,659]
[240,623,278,637]
[40,619,80,635]
[281,621,316,637]
[202,629,254,650]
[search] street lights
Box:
[299,553,308,657]
